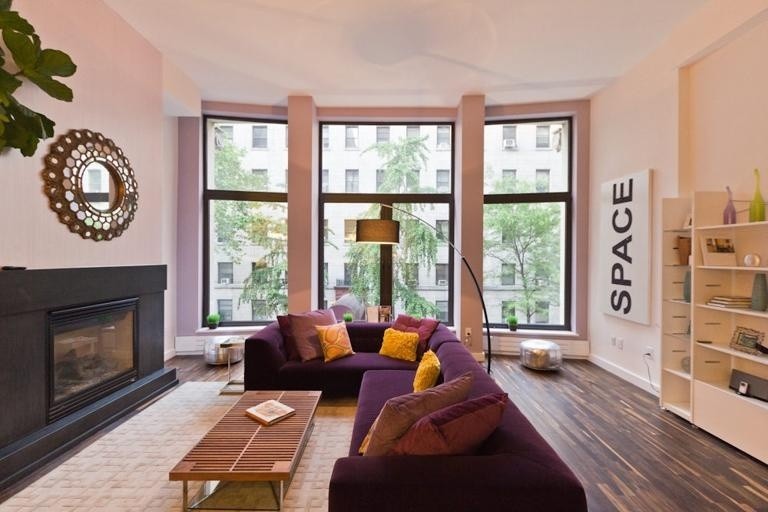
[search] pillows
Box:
[412,350,441,393]
[276,307,338,362]
[379,328,419,363]
[392,314,440,359]
[316,319,354,363]
[358,372,510,457]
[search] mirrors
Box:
[41,127,140,243]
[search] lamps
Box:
[354,205,492,374]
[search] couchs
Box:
[243,318,589,512]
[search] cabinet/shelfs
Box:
[656,188,768,468]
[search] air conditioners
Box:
[438,280,447,287]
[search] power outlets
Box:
[645,345,657,361]
[465,328,471,337]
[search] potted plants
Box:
[506,316,518,331]
[343,312,354,324]
[205,313,221,329]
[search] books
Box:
[245,399,297,427]
[379,306,391,324]
[367,307,378,322]
[706,295,751,309]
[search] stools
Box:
[205,341,245,365]
[519,339,564,372]
